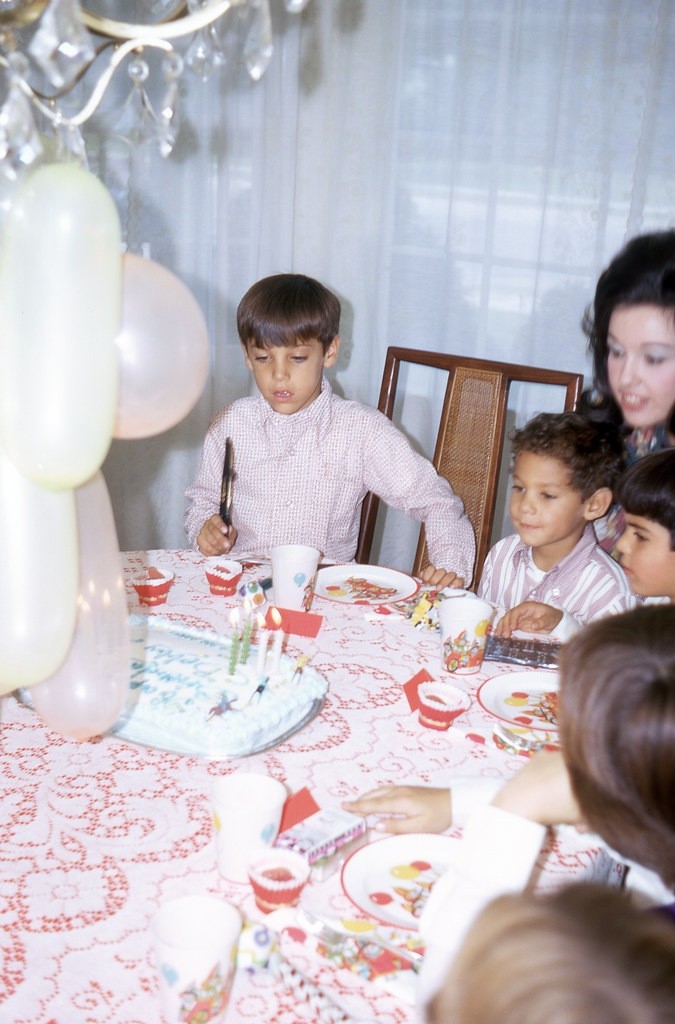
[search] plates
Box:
[313,564,420,605]
[341,833,462,931]
[477,669,561,731]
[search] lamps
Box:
[1,1,308,178]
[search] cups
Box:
[270,544,321,614]
[437,597,493,675]
[210,773,288,885]
[149,893,243,1024]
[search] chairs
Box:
[355,345,584,596]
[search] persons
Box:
[476,414,636,644]
[491,447,674,643]
[577,227,675,601]
[423,881,675,1024]
[339,603,675,1024]
[183,274,476,590]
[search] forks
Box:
[296,908,423,968]
[493,726,544,752]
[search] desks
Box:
[0,549,663,1024]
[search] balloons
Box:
[3,163,125,489]
[29,468,133,739]
[112,251,211,440]
[0,469,80,696]
